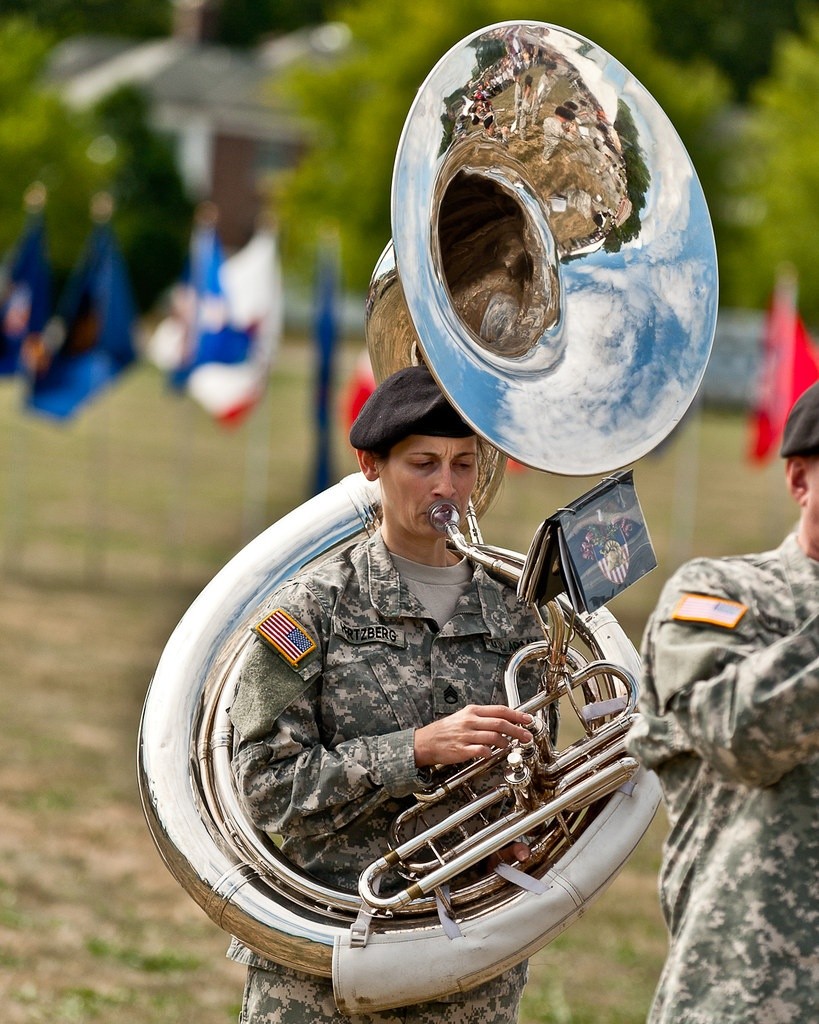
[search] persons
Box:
[623,377,819,1024]
[227,362,644,1024]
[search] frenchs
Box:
[136,21,723,1011]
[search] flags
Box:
[21,224,138,418]
[744,289,819,464]
[339,350,375,429]
[0,212,49,376]
[147,234,285,425]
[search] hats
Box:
[349,365,476,453]
[781,379,819,459]
